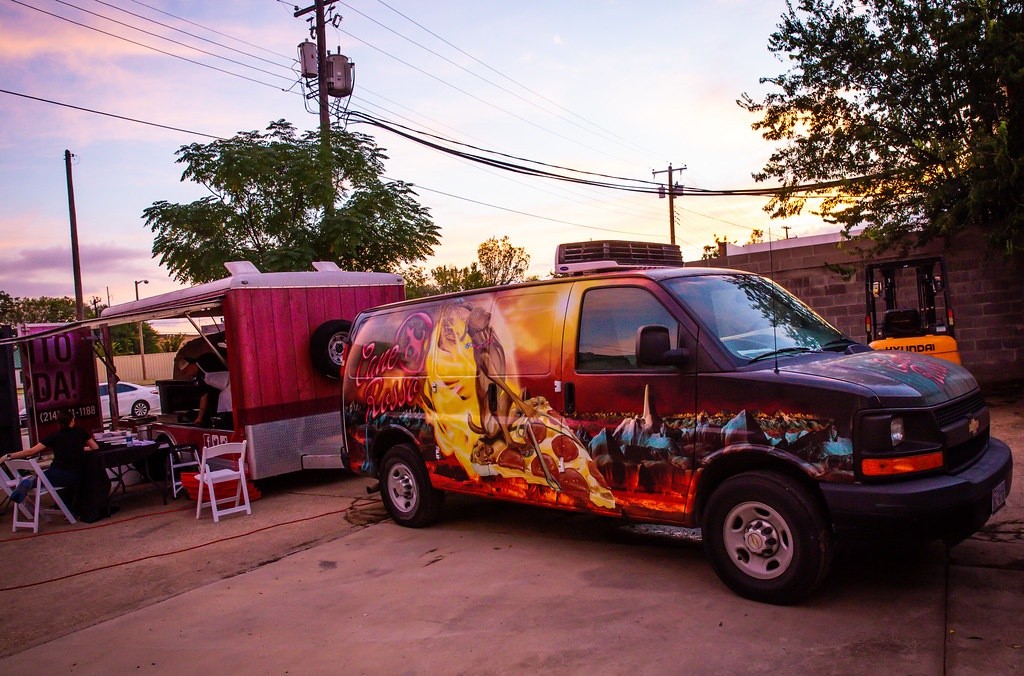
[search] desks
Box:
[83,437,168,516]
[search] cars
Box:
[19,382,161,427]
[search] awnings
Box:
[0,300,229,378]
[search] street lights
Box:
[135,279,149,381]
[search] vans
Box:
[339,239,1014,605]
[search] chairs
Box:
[4,458,76,533]
[170,446,202,499]
[882,308,918,339]
[0,467,33,520]
[194,440,251,523]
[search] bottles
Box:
[126,428,133,447]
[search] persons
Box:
[176,352,224,425]
[0,410,99,521]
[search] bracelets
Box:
[6,453,11,460]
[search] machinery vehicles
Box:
[864,254,962,369]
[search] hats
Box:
[177,357,198,371]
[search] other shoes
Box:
[64,512,80,522]
[9,475,37,504]
